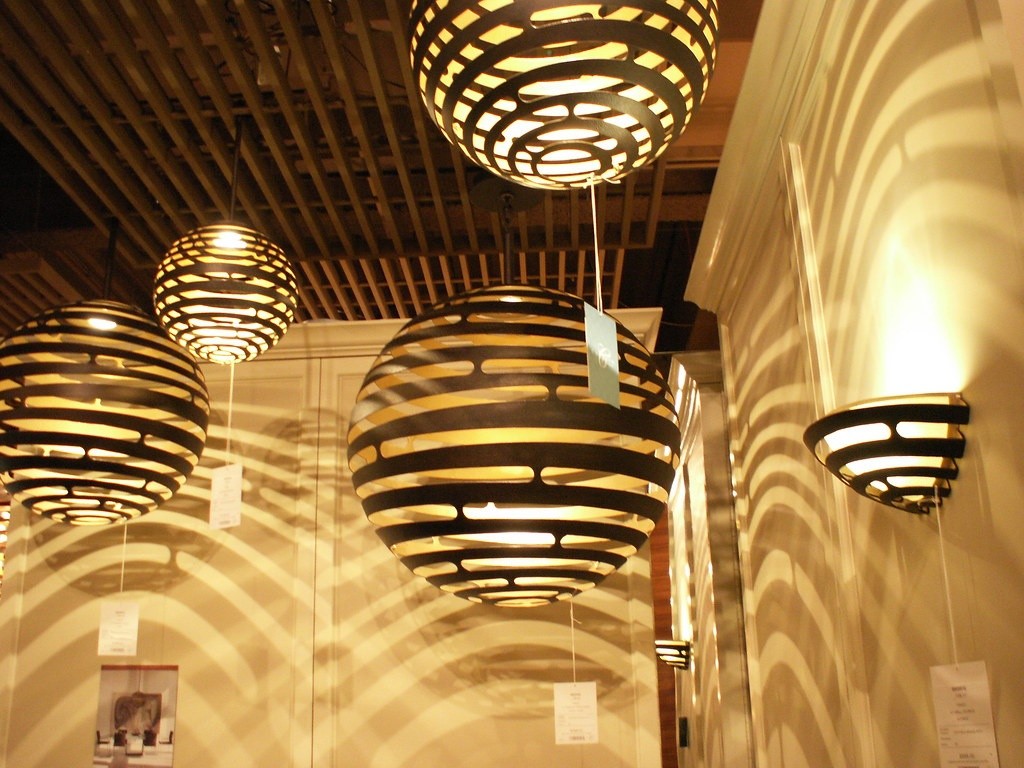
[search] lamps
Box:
[407,0,720,189]
[803,390,965,514]
[0,216,213,526]
[654,638,694,669]
[150,118,298,366]
[345,165,701,601]
